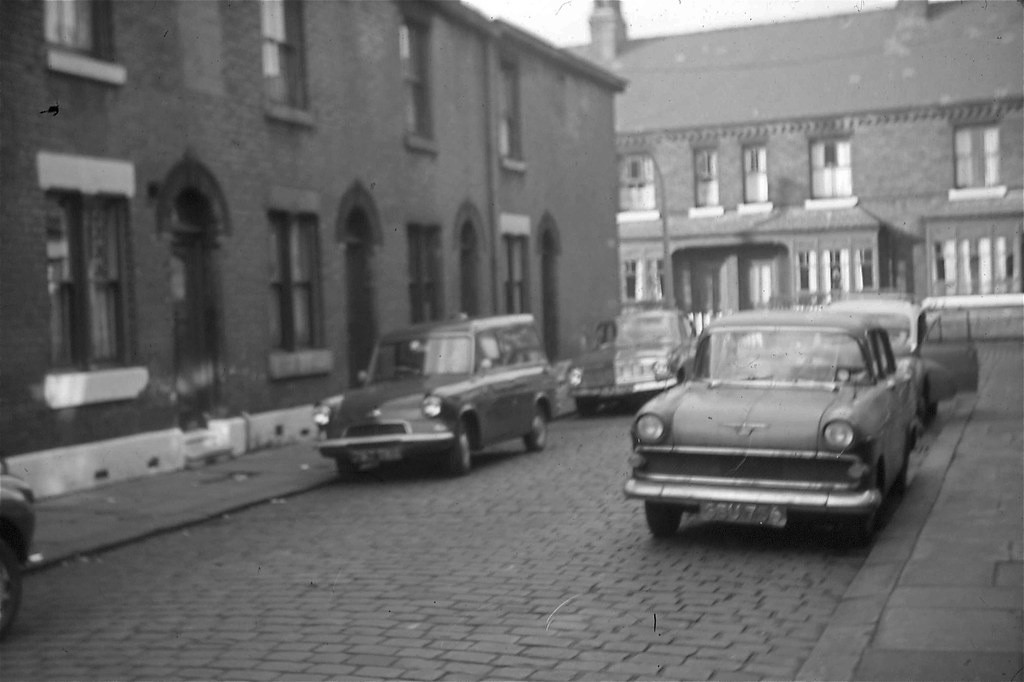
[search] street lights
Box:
[628,151,673,301]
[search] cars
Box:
[564,310,696,415]
[0,459,36,637]
[312,312,558,479]
[823,300,979,429]
[618,300,697,338]
[625,310,917,541]
